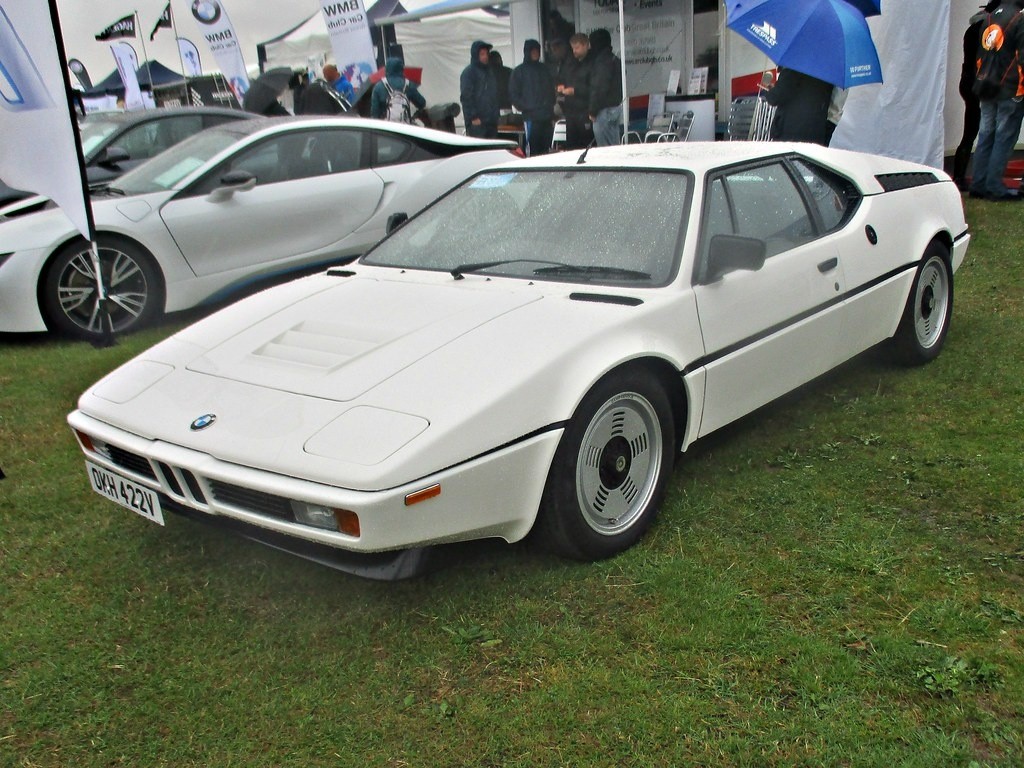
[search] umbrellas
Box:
[243,67,292,113]
[724,1,883,88]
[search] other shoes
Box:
[969,188,1021,201]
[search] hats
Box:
[481,43,493,50]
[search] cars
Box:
[0,105,530,345]
[66,139,972,580]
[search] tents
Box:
[69,57,240,110]
[375,0,947,174]
[257,0,513,146]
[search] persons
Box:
[758,68,850,148]
[296,30,623,155]
[947,0,1024,202]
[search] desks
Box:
[665,92,715,142]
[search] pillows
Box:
[499,114,508,125]
[508,114,523,126]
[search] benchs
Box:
[497,125,525,146]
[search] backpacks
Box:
[381,76,411,125]
[971,11,1021,101]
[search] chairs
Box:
[726,96,778,141]
[271,135,310,181]
[621,113,675,144]
[551,119,566,148]
[309,135,358,174]
[645,113,695,143]
